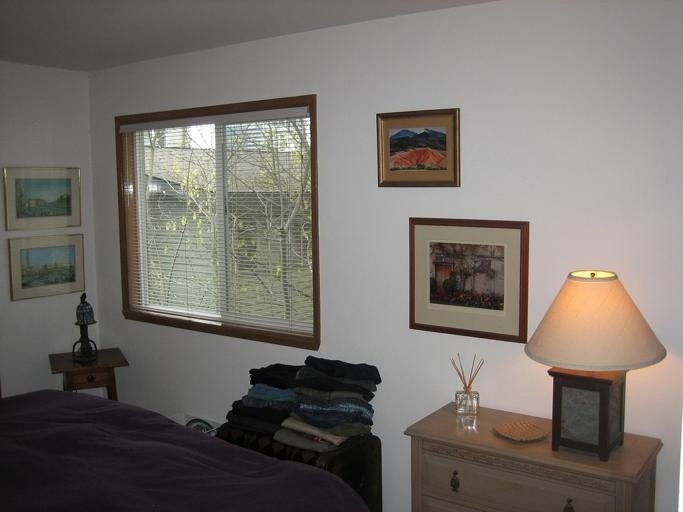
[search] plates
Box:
[493,420,549,442]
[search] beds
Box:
[1,389,370,512]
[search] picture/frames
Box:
[407,216,529,345]
[2,164,82,231]
[375,106,461,188]
[7,233,85,302]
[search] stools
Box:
[214,420,382,512]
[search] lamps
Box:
[524,270,666,462]
[71,293,98,362]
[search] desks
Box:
[47,347,129,403]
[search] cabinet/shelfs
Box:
[404,401,664,511]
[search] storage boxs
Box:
[167,413,222,437]
